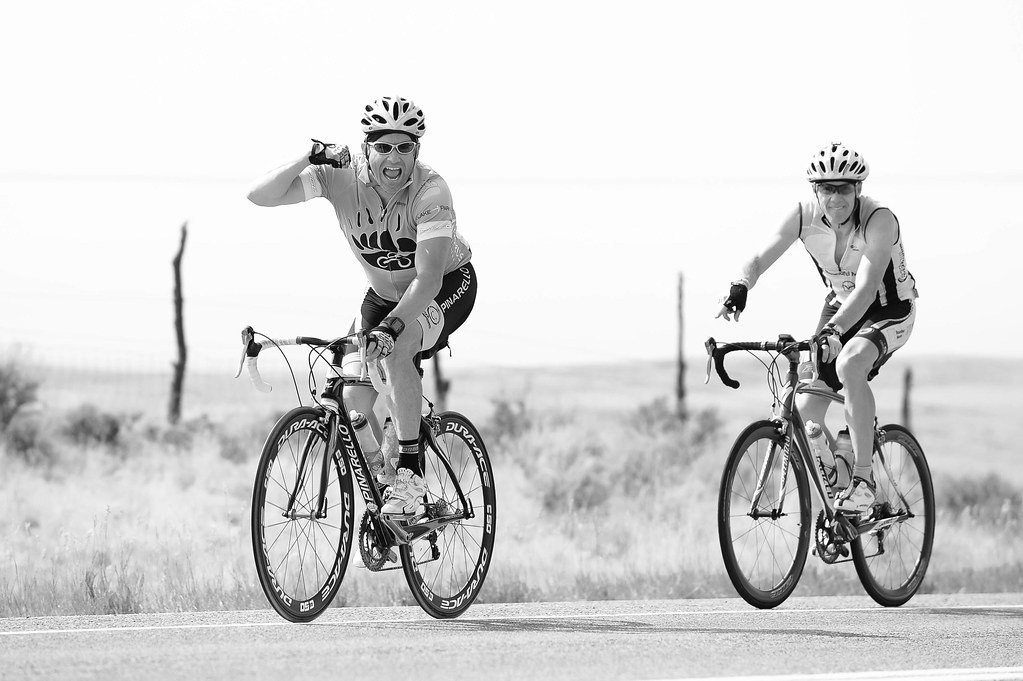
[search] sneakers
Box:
[381,468,427,516]
[812,527,843,557]
[832,475,878,513]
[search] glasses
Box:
[367,141,418,155]
[815,183,858,195]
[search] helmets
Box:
[806,143,869,182]
[361,96,426,138]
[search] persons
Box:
[716,143,917,514]
[248,96,478,518]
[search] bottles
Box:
[377,417,399,485]
[833,425,853,489]
[348,409,384,467]
[804,419,838,485]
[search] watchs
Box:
[825,323,844,336]
[381,317,406,335]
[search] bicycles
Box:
[702,334,937,609]
[236,326,496,624]
